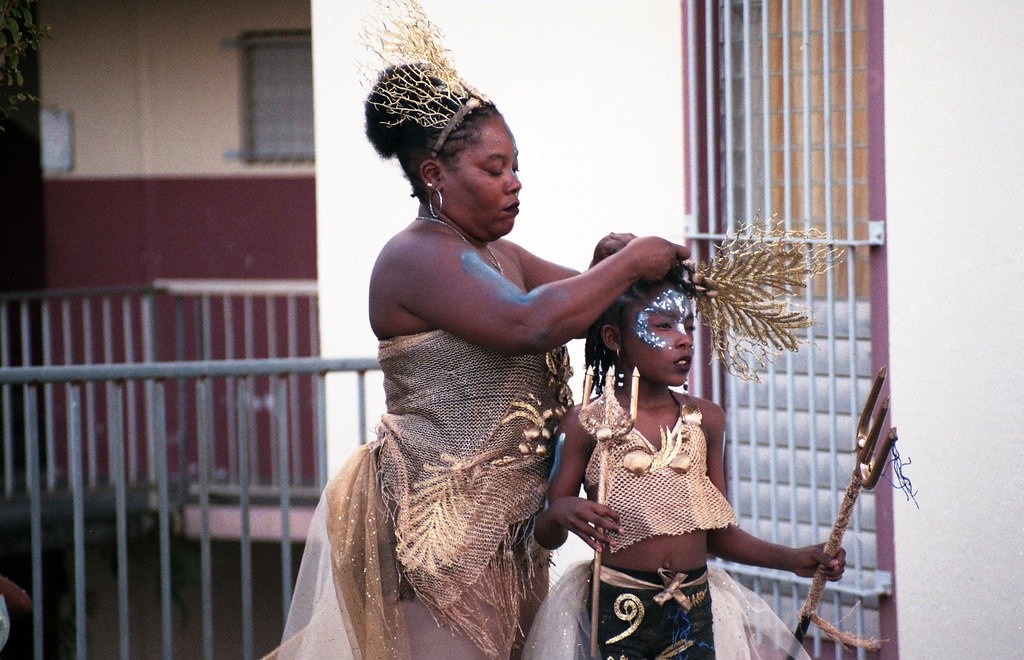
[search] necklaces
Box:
[416,217,503,275]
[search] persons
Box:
[520,246,846,660]
[306,64,691,660]
